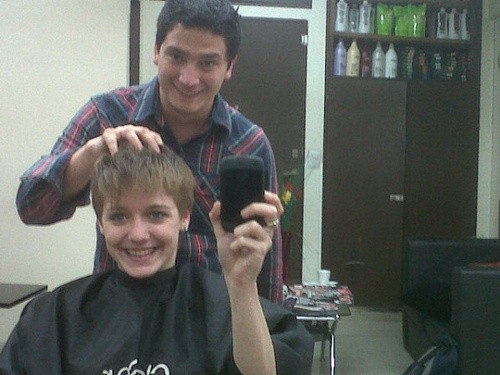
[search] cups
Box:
[318,270,331,285]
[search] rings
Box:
[263,219,280,228]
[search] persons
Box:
[0,141,316,375]
[15,0,283,309]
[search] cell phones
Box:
[219,155,265,235]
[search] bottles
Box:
[335,37,472,82]
[335,0,474,40]
[437,8,471,40]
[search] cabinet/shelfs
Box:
[217,0,483,312]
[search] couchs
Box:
[402,237,500,375]
